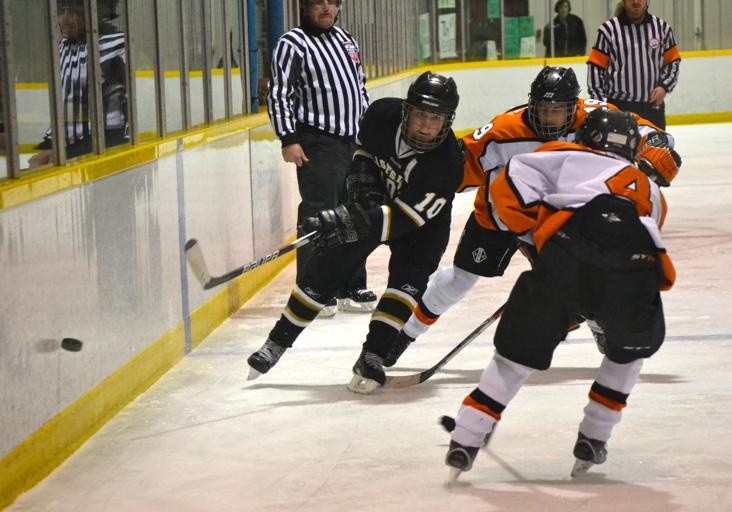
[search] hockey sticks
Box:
[184,231,324,288]
[383,302,508,388]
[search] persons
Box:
[445,106,675,474]
[246,72,465,386]
[586,0,681,130]
[29,0,133,165]
[384,69,669,368]
[543,2,587,57]
[267,0,376,306]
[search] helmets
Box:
[401,71,459,152]
[57,0,120,37]
[528,66,640,161]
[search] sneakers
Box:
[446,439,479,470]
[336,285,376,302]
[248,335,286,373]
[574,431,606,463]
[353,330,415,386]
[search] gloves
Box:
[637,130,680,186]
[302,162,385,251]
